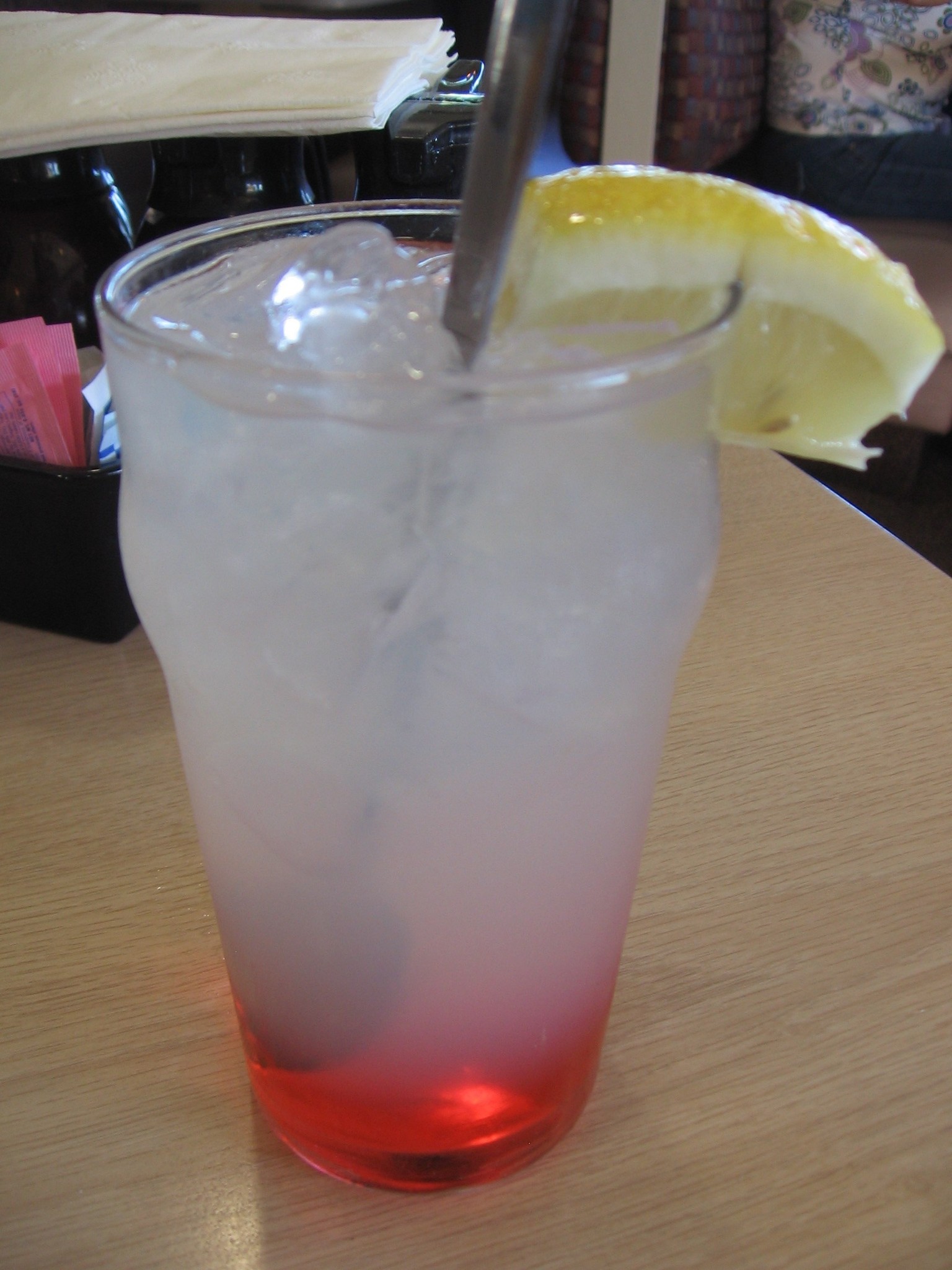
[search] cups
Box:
[93,200,752,1195]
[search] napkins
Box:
[1,10,458,158]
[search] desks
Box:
[0,345,952,1270]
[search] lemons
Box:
[478,163,945,472]
